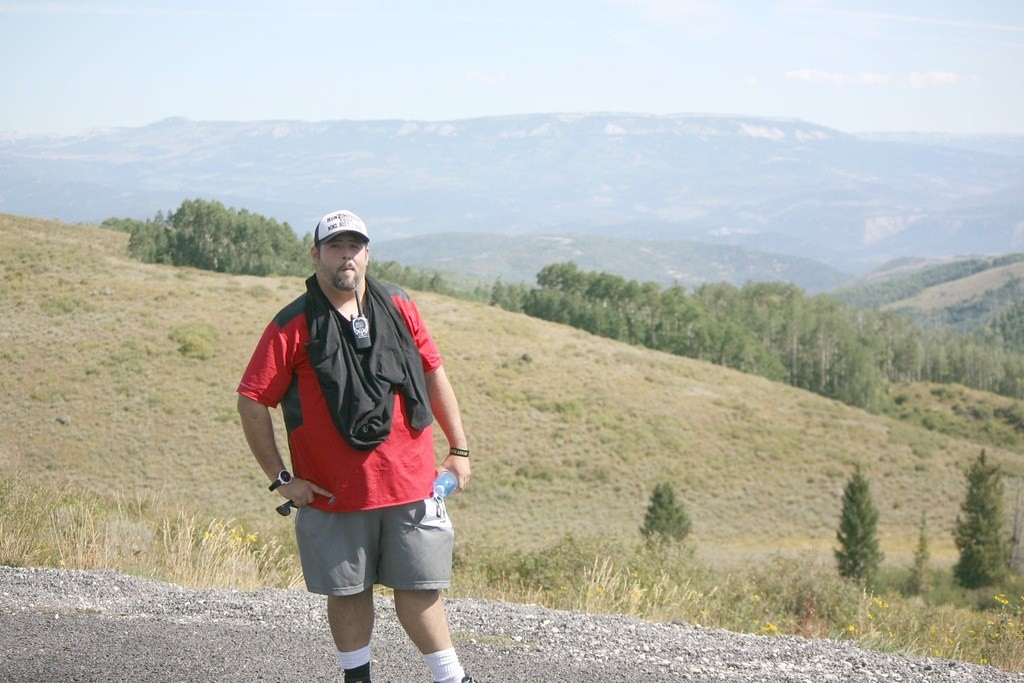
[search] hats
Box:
[314,211,370,247]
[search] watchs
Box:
[268,469,292,492]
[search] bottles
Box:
[434,471,459,498]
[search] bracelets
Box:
[449,447,469,457]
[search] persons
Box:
[235,209,474,683]
